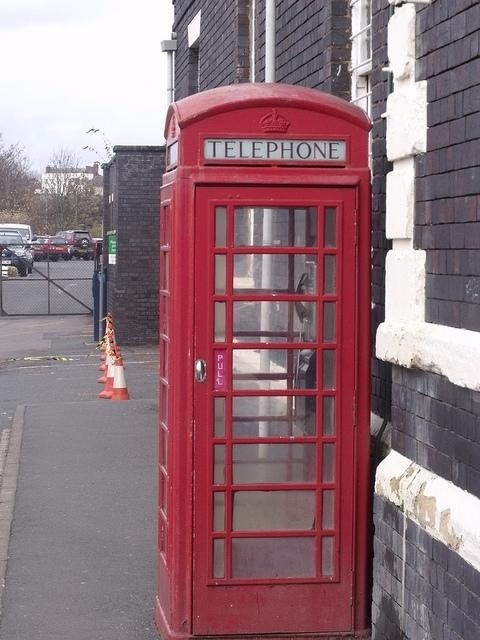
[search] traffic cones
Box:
[96,311,129,400]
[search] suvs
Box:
[32,235,69,262]
[57,229,94,260]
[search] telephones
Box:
[294,263,316,324]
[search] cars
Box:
[0,223,37,277]
